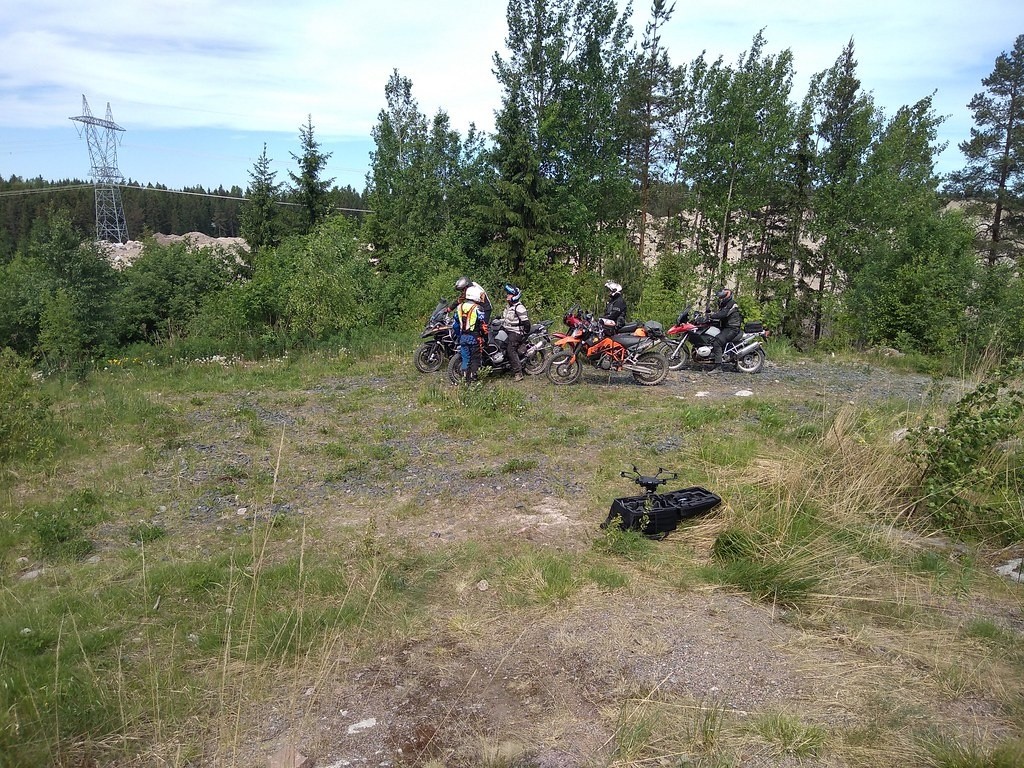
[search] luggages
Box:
[601,481,722,540]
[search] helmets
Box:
[715,288,733,305]
[504,283,521,303]
[464,285,484,304]
[454,276,475,291]
[604,281,623,298]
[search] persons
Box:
[500,284,530,382]
[454,286,489,381]
[707,289,744,375]
[599,282,628,335]
[444,276,493,324]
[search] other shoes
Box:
[515,371,525,382]
[708,365,726,375]
[464,372,478,383]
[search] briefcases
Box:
[743,321,764,333]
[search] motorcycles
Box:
[413,294,504,374]
[656,301,771,375]
[546,313,669,386]
[447,315,552,387]
[541,303,644,361]
